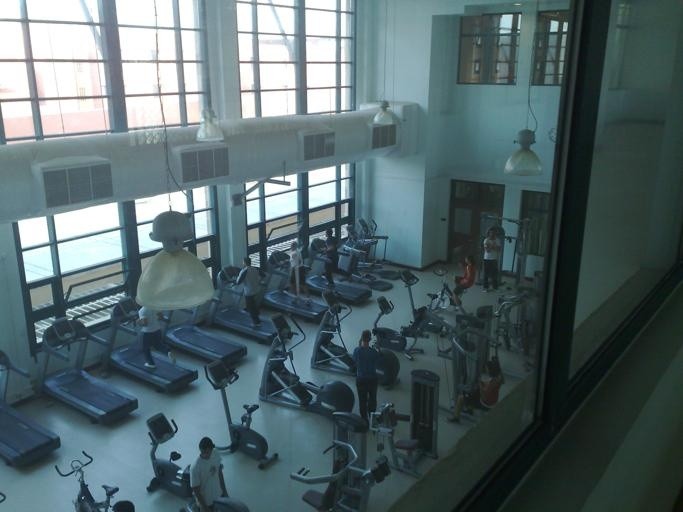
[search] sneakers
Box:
[289,299,314,308]
[324,281,337,287]
[250,322,265,327]
[144,363,157,369]
[168,351,177,366]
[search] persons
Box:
[449,254,475,306]
[446,359,501,423]
[351,330,379,420]
[111,500,135,512]
[285,240,313,307]
[189,437,229,512]
[135,305,176,369]
[345,225,360,249]
[233,258,266,328]
[480,228,501,294]
[319,227,338,286]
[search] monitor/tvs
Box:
[322,288,339,309]
[377,296,392,314]
[52,317,76,340]
[270,312,291,336]
[207,359,229,387]
[222,264,237,280]
[146,412,175,443]
[119,296,139,317]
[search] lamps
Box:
[196,1,224,142]
[134,1,214,309]
[505,0,543,174]
[373,0,400,126]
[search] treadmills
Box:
[0,350,60,468]
[263,251,329,323]
[34,317,138,424]
[100,297,198,394]
[203,266,279,345]
[304,239,372,304]
[159,306,247,366]
